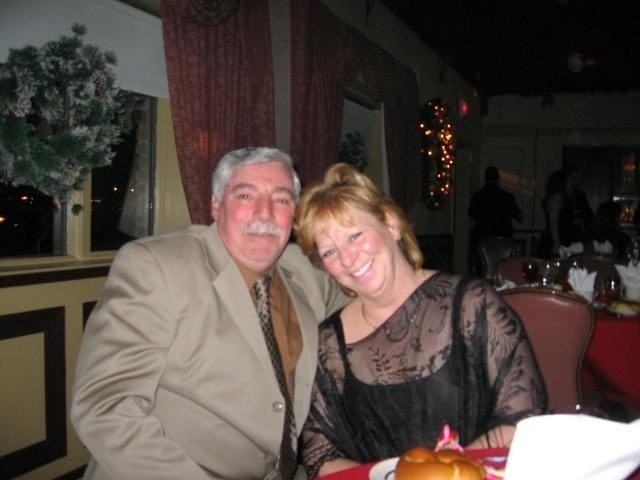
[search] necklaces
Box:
[362,268,426,335]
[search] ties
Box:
[253,274,299,479]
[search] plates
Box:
[368,455,400,479]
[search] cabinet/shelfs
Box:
[563,145,640,244]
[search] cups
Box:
[603,274,625,312]
[521,256,539,288]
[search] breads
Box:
[395,446,488,480]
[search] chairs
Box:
[497,287,595,414]
[479,235,513,284]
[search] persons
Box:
[469,166,524,277]
[71,144,349,479]
[537,167,640,271]
[294,162,548,480]
